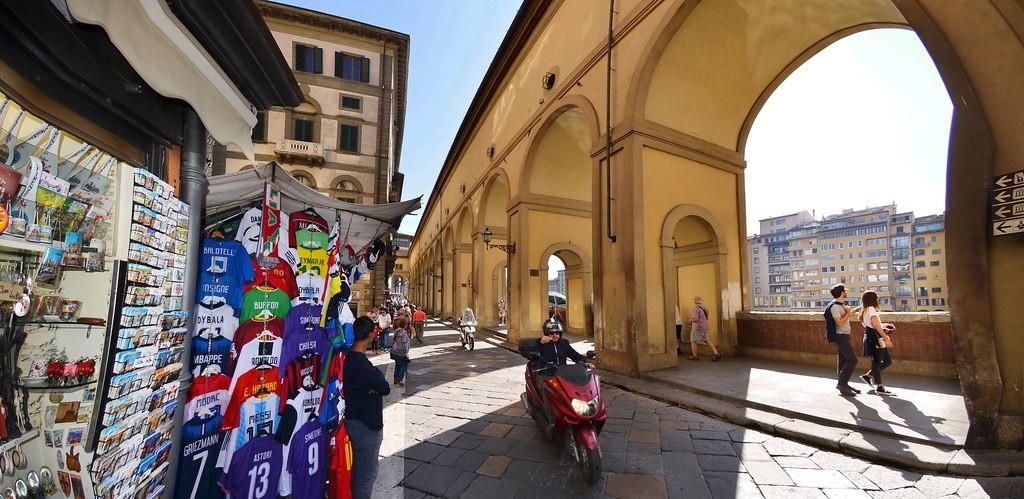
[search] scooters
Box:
[517,345,608,484]
[458,316,479,351]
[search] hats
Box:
[693,295,704,303]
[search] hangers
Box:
[191,203,321,440]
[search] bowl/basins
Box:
[62,304,78,312]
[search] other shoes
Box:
[840,386,861,394]
[546,419,555,433]
[689,355,699,360]
[417,340,419,342]
[419,339,423,343]
[394,378,404,386]
[860,375,875,389]
[875,386,893,395]
[836,383,857,396]
[712,354,721,361]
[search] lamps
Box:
[482,227,516,253]
[409,268,443,289]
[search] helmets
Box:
[543,318,563,336]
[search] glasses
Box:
[548,331,560,335]
[381,312,384,313]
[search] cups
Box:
[0,450,14,474]
[28,359,50,378]
[12,443,26,467]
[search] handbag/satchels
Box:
[390,353,395,359]
[876,336,890,348]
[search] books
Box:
[91,153,190,498]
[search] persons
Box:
[413,306,427,344]
[859,291,894,394]
[826,284,862,396]
[461,307,477,335]
[689,295,721,361]
[342,316,390,499]
[372,309,391,355]
[385,300,416,338]
[535,318,595,427]
[390,318,410,386]
[676,306,685,357]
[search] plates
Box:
[21,377,49,386]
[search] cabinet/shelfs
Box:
[6,265,109,389]
